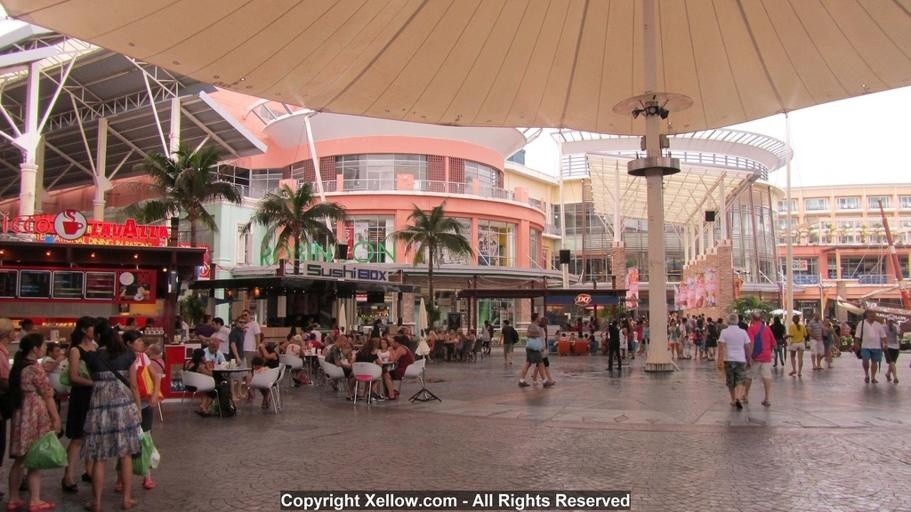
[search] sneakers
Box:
[543,381,552,386]
[519,381,530,387]
[143,477,154,489]
[735,398,743,410]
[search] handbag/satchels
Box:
[751,334,763,358]
[855,338,862,360]
[136,366,163,401]
[526,336,545,352]
[60,359,92,387]
[512,329,520,343]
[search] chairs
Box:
[153,311,642,422]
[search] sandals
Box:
[28,500,55,512]
[7,499,25,510]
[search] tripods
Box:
[409,355,441,404]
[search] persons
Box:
[0,308,517,511]
[518,302,911,411]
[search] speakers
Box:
[705,211,715,221]
[159,270,178,294]
[560,250,570,263]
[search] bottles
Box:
[145,327,164,335]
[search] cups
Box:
[317,348,322,355]
[62,221,84,235]
[230,359,236,369]
[311,348,316,356]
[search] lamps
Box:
[614,90,691,120]
